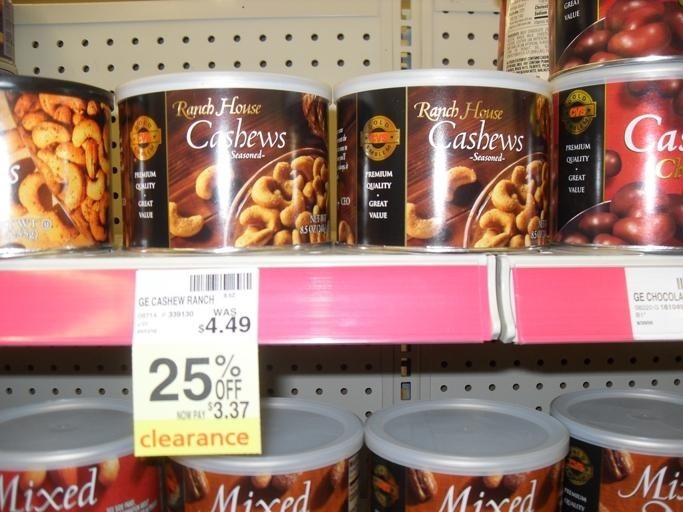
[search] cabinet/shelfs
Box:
[0,0,683,511]
